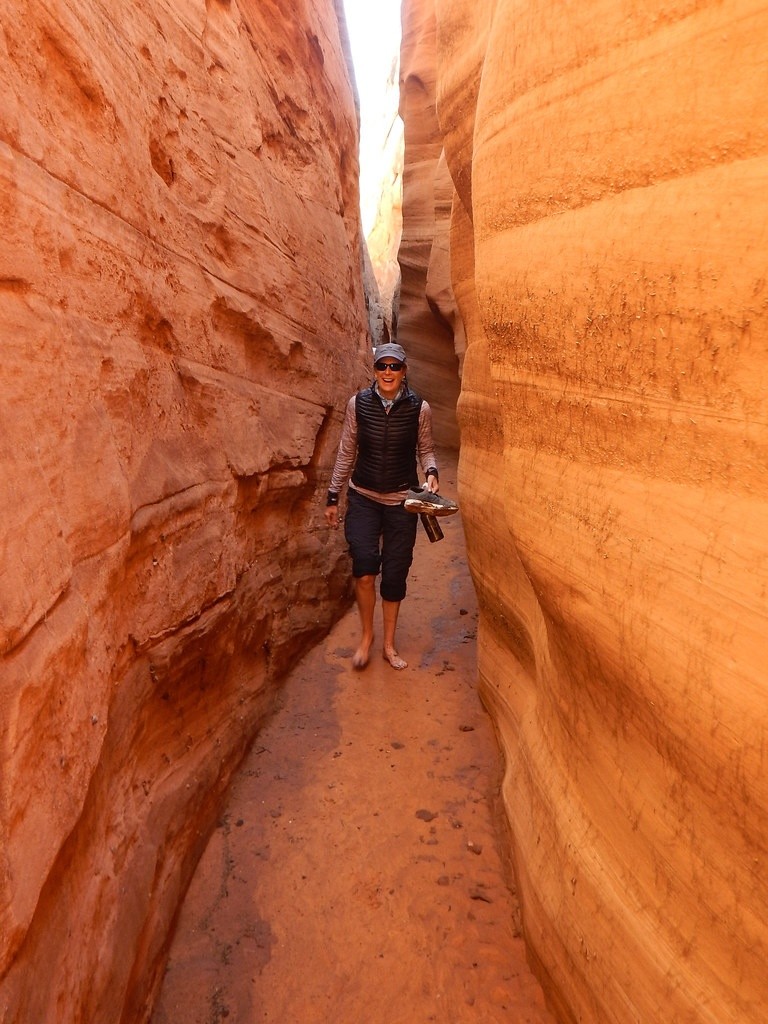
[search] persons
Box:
[324,343,439,670]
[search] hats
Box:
[373,343,408,369]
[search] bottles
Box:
[419,512,444,544]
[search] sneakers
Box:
[404,488,459,516]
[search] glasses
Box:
[376,363,404,371]
